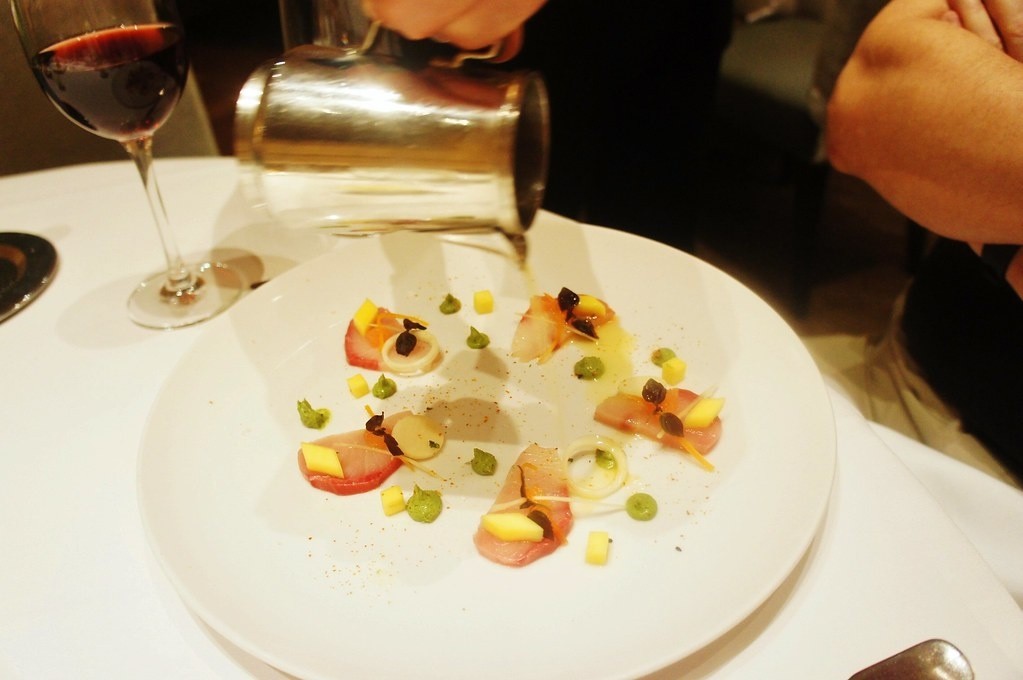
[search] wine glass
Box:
[10,0,243,329]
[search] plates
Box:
[138,213,837,680]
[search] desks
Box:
[0,156,1023,680]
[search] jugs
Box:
[231,21,553,271]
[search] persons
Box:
[151,0,734,256]
[827,0,1023,489]
